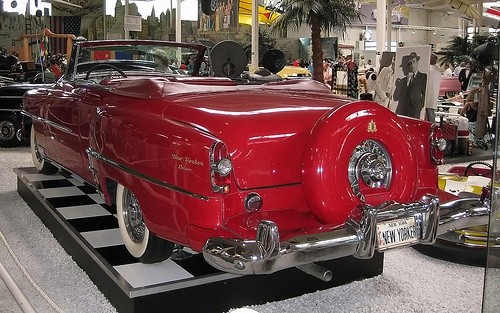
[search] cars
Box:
[0,54,182,148]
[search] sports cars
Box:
[20,37,447,276]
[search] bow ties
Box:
[465,68,470,71]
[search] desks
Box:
[437,116,469,155]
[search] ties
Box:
[411,74,416,85]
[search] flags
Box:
[37,31,49,62]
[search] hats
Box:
[406,52,421,66]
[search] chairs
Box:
[34,72,55,83]
[439,122,458,158]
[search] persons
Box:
[398,52,427,119]
[443,64,452,77]
[291,54,374,94]
[374,51,396,108]
[153,49,173,73]
[425,52,442,122]
[50,58,62,81]
[459,62,481,104]
